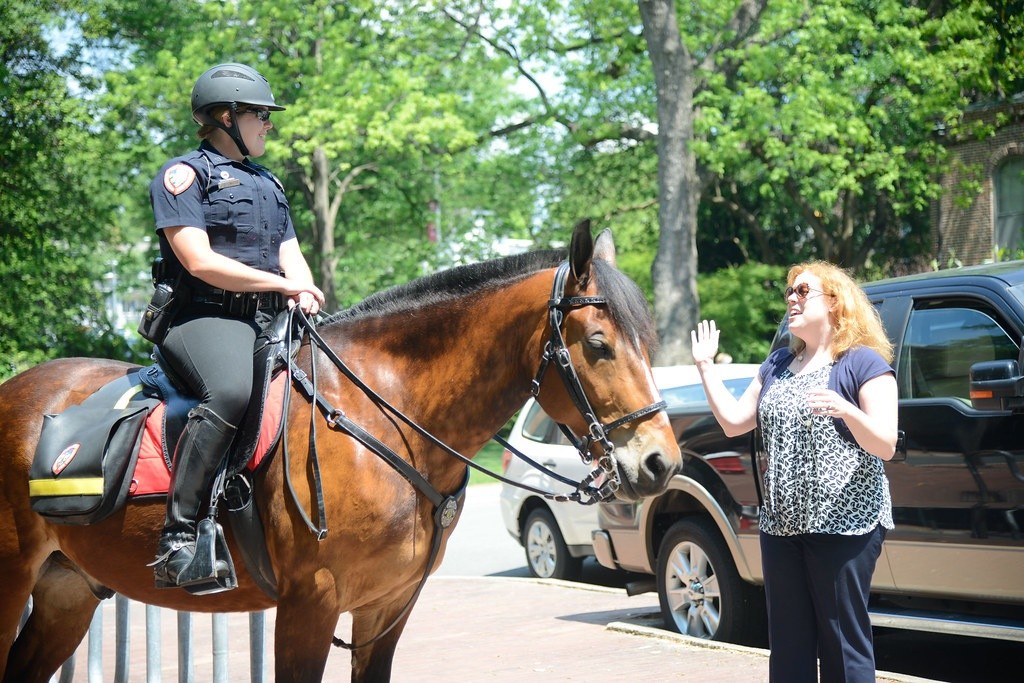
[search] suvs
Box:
[594,259,1024,644]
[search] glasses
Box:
[236,108,271,122]
[785,283,833,304]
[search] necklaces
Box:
[795,351,832,372]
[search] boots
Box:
[155,407,238,588]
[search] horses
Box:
[1,219,684,683]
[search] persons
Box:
[136,58,324,590]
[689,256,901,683]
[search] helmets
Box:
[191,63,286,127]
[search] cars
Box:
[502,361,764,581]
